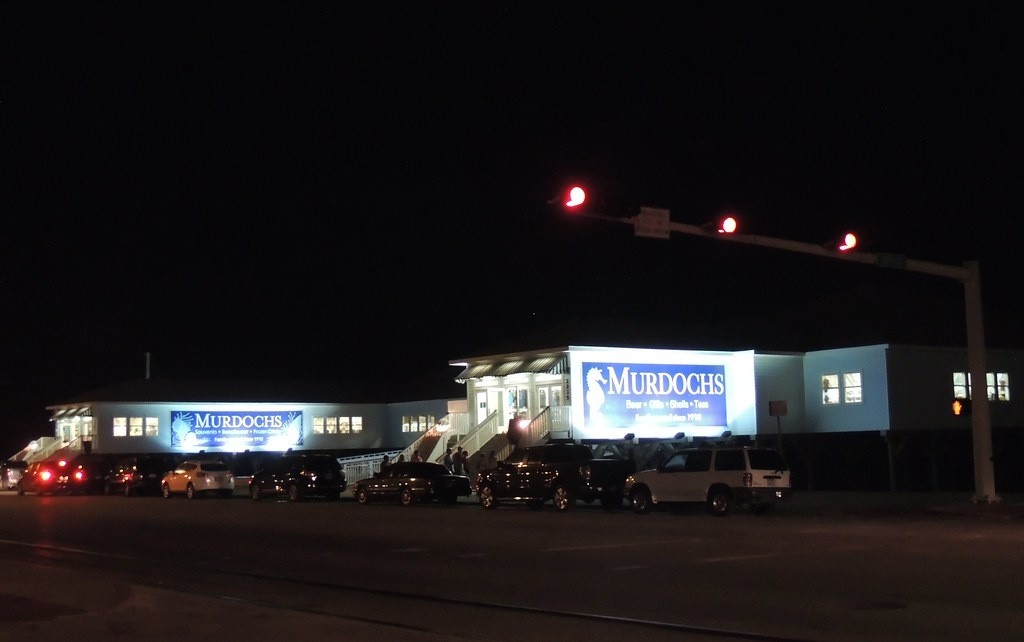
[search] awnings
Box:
[454,355,568,384]
[49,407,92,421]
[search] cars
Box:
[160,459,235,499]
[351,461,472,505]
[103,458,173,497]
[17,461,92,497]
[0,460,29,491]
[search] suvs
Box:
[248,453,348,503]
[623,446,792,517]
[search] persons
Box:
[380,450,422,472]
[444,446,498,476]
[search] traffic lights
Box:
[951,398,971,417]
[564,184,588,212]
[719,217,740,235]
[837,234,857,255]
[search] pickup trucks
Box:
[474,443,626,513]
